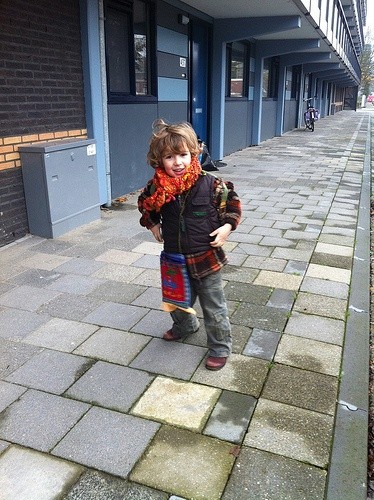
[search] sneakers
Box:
[207,355,227,370]
[164,328,200,340]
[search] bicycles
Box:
[302,95,319,132]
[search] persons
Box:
[137,117,242,371]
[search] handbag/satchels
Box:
[159,251,191,308]
